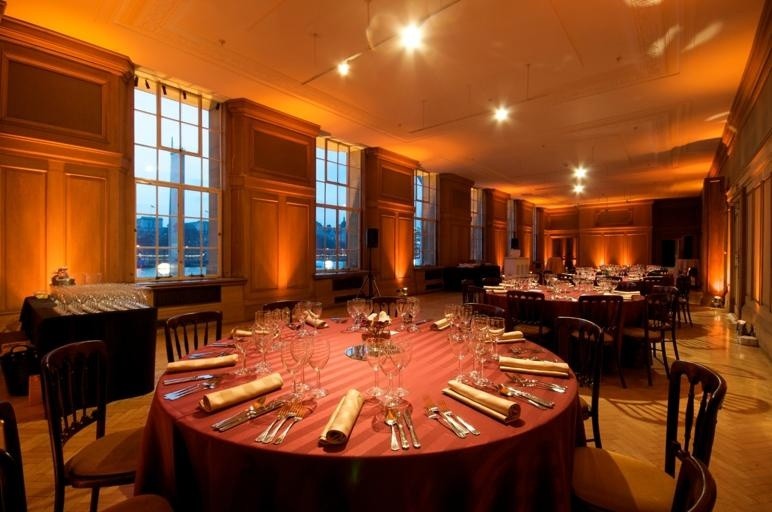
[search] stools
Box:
[100,494,170,512]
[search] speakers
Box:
[511,238,519,249]
[366,228,378,248]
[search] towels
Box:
[230,326,271,336]
[318,389,364,449]
[200,373,285,412]
[442,378,521,425]
[430,317,454,331]
[166,354,239,373]
[303,309,329,329]
[378,310,392,327]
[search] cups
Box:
[33,290,49,299]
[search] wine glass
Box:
[51,283,153,315]
[499,269,619,300]
[230,295,507,406]
[575,263,664,281]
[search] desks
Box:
[20,291,158,419]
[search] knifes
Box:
[212,399,289,433]
[403,408,421,449]
[165,374,215,386]
[496,380,555,410]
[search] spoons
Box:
[385,406,400,451]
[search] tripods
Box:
[357,248,381,298]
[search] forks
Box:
[162,379,229,401]
[504,368,568,396]
[422,395,481,439]
[394,408,409,449]
[252,399,313,445]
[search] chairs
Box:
[667,445,718,509]
[1,402,30,511]
[368,296,415,319]
[450,260,726,389]
[43,339,146,512]
[567,359,726,510]
[165,308,222,371]
[552,314,610,450]
[261,300,306,326]
